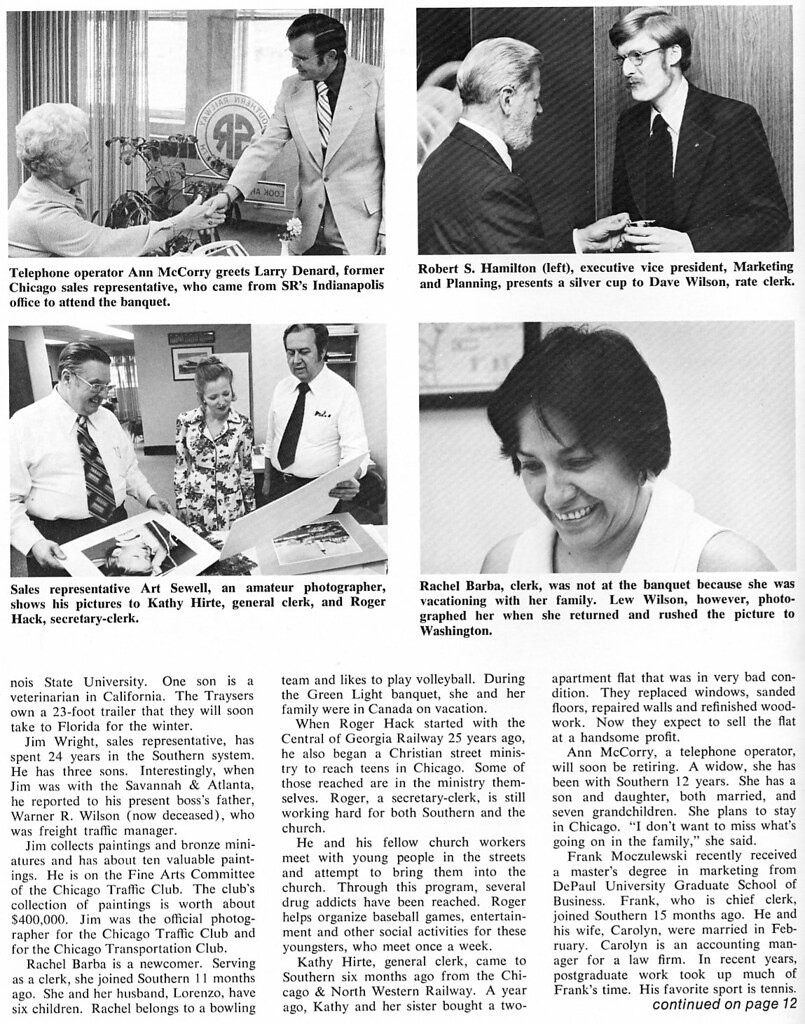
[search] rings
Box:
[208,221,214,225]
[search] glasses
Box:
[68,368,117,391]
[612,46,664,66]
[284,48,326,63]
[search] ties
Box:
[650,114,673,221]
[276,381,311,470]
[76,414,116,522]
[316,81,332,148]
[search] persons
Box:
[262,324,370,501]
[200,15,386,255]
[105,526,168,576]
[175,357,256,533]
[611,8,791,252]
[481,325,778,572]
[418,38,630,255]
[7,102,227,258]
[9,342,175,577]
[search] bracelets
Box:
[220,191,231,210]
[168,218,180,237]
